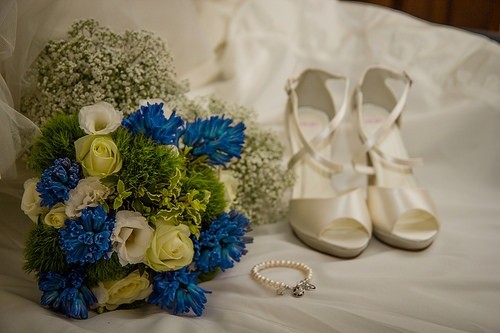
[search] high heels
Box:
[284,65,439,259]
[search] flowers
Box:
[21,98,254,320]
[21,18,298,227]
